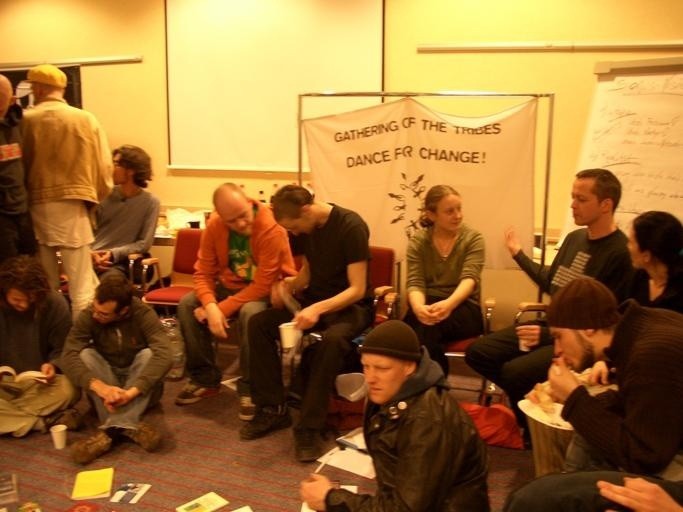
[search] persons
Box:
[504,469,682,511]
[400,184,484,376]
[91,144,162,276]
[464,168,631,450]
[614,211,683,312]
[16,63,115,325]
[57,269,175,464]
[0,255,80,438]
[298,320,491,511]
[0,74,37,257]
[174,183,298,420]
[545,277,682,476]
[239,184,376,461]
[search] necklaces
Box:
[433,232,456,262]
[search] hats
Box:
[363,320,423,360]
[547,277,620,329]
[24,64,68,88]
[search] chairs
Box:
[213,253,303,371]
[289,246,402,393]
[383,292,495,406]
[513,302,548,323]
[56,252,155,297]
[141,228,202,315]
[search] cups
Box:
[519,339,532,352]
[279,322,301,348]
[186,221,200,229]
[50,425,68,450]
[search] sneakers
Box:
[176,382,219,405]
[294,426,319,461]
[238,391,256,421]
[74,431,112,463]
[122,422,160,451]
[240,404,292,439]
[46,408,81,432]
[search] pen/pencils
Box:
[315,452,332,474]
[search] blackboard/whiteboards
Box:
[547,56,681,250]
[164,0,385,173]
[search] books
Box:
[1,473,19,504]
[316,446,378,480]
[109,483,152,503]
[176,491,230,512]
[0,365,48,384]
[335,427,368,452]
[70,467,115,500]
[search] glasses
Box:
[87,303,110,319]
